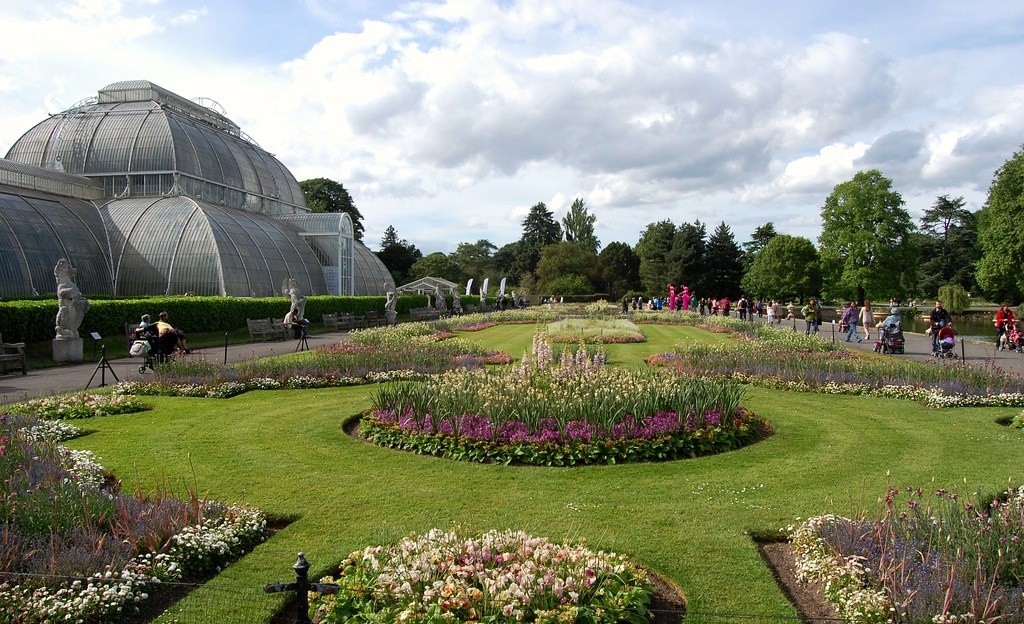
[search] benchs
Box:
[322,312,351,333]
[246,318,287,343]
[272,317,305,340]
[341,312,365,332]
[125,322,140,358]
[0,331,27,376]
[410,303,497,321]
[364,310,388,327]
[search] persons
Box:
[283,307,309,339]
[995,301,1015,350]
[881,306,902,345]
[495,296,527,312]
[930,300,953,356]
[137,311,193,359]
[889,297,928,309]
[449,305,458,318]
[539,293,565,305]
[621,291,877,344]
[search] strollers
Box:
[999,318,1024,354]
[934,322,959,360]
[837,318,848,334]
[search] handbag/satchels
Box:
[130,343,147,357]
[802,307,808,317]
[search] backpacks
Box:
[741,300,748,308]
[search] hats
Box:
[890,307,899,314]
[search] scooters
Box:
[873,321,906,355]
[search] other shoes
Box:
[294,336,301,339]
[931,352,935,356]
[995,346,999,350]
[304,335,311,338]
[858,339,862,343]
[185,347,194,355]
[846,339,853,342]
